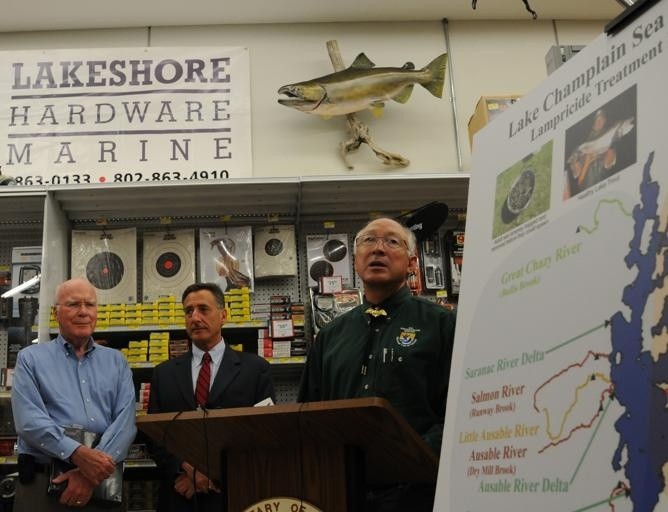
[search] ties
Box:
[192,352,215,411]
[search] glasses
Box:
[56,300,97,309]
[356,232,411,254]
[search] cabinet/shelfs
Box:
[48,313,308,472]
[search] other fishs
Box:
[275,48,449,119]
[566,116,634,158]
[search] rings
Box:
[76,500,82,504]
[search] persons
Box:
[298,217,456,512]
[144,281,274,512]
[9,280,138,512]
[562,108,618,200]
